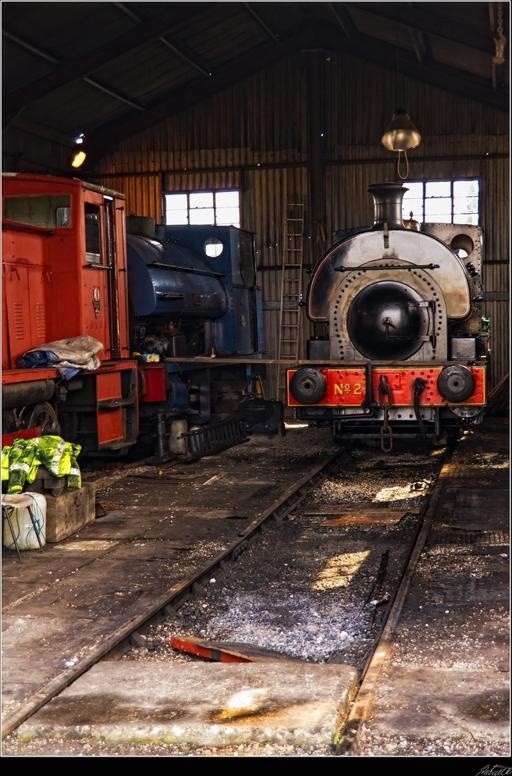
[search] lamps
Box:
[62,148,86,169]
[380,1,422,152]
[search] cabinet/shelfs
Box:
[3,171,143,456]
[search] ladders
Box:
[178,416,251,464]
[276,194,307,420]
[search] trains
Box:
[1,172,266,455]
[285,183,486,451]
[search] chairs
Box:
[1,488,44,559]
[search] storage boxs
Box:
[35,482,97,543]
